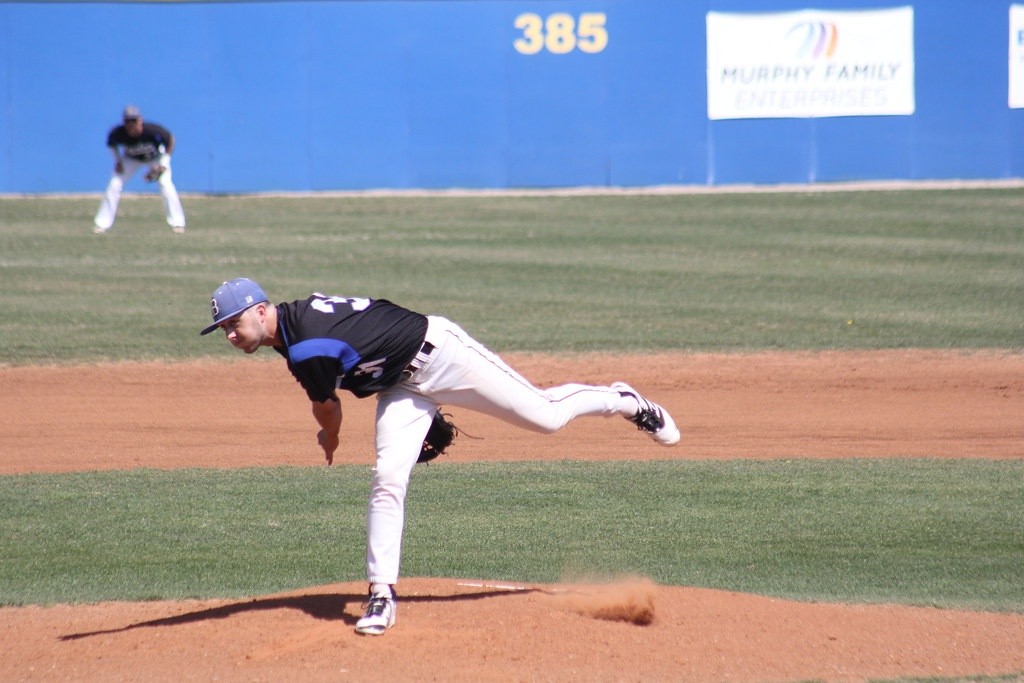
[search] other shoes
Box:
[92,226,105,235]
[172,226,185,235]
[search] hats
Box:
[201,278,269,335]
[122,108,140,119]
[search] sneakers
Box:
[355,589,396,635]
[611,383,680,447]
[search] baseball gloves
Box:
[413,405,455,464]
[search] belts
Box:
[399,342,435,383]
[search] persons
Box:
[200,277,680,636]
[91,107,185,235]
